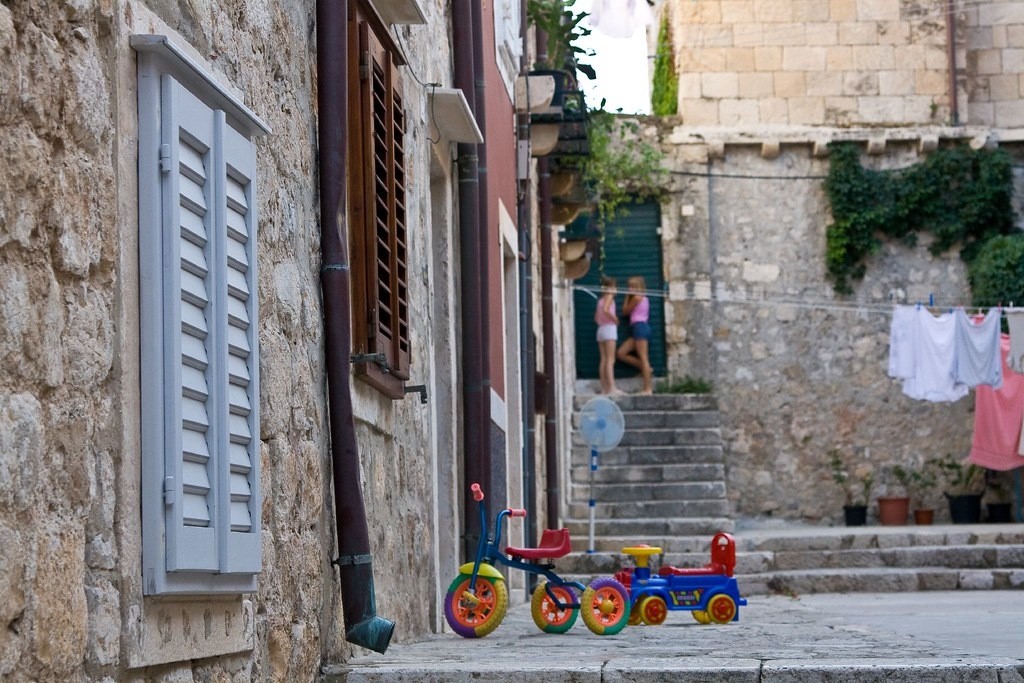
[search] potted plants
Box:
[913,470,936,525]
[828,448,872,526]
[987,485,1014,523]
[876,496,911,526]
[938,453,985,526]
[526,0,671,286]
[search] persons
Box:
[594,276,624,396]
[617,276,654,396]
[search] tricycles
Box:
[444,483,632,640]
